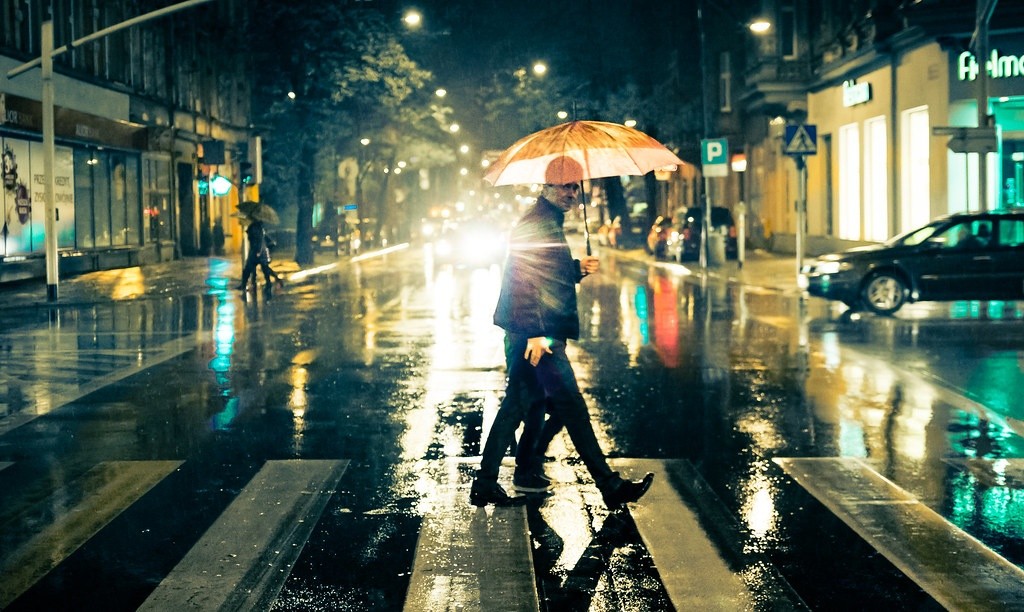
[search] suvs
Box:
[664,203,737,264]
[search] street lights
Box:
[735,18,772,271]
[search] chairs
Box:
[976,224,990,245]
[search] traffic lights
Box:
[240,161,253,185]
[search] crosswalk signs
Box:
[783,124,820,156]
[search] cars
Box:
[311,216,361,257]
[645,214,667,261]
[799,209,1024,319]
[357,218,387,251]
[596,212,649,251]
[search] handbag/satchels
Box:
[265,234,277,250]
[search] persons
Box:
[469,178,655,511]
[954,227,979,247]
[235,214,284,289]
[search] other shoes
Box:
[235,284,245,290]
[263,282,273,291]
[511,468,557,492]
[603,471,655,510]
[246,285,257,292]
[469,479,531,507]
[279,279,284,289]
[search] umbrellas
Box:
[235,201,281,226]
[482,100,684,257]
